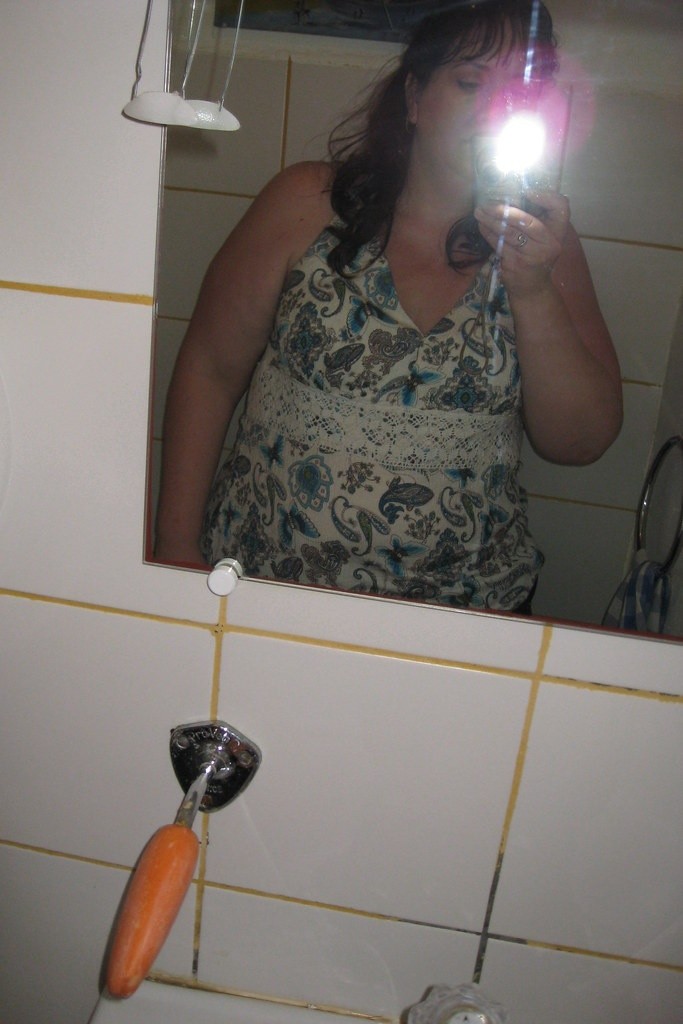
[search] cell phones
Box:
[471,132,528,217]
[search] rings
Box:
[518,234,528,247]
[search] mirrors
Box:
[138,0,683,647]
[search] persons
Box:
[158,0,625,615]
